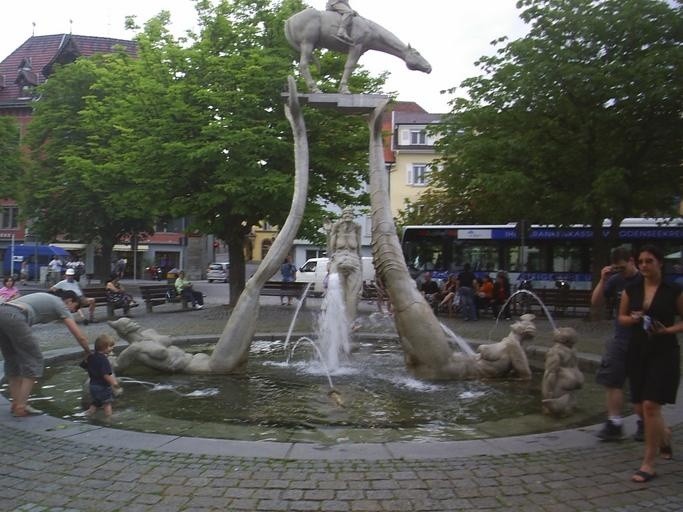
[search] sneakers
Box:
[595,419,626,440]
[192,303,208,310]
[632,420,645,442]
[9,402,43,417]
[491,316,511,321]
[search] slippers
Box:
[632,470,656,482]
[660,424,673,460]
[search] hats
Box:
[65,268,75,276]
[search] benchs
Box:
[134,280,208,316]
[78,285,140,321]
[414,283,593,323]
[255,278,315,308]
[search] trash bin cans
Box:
[39,266,48,282]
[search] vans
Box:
[293,256,385,299]
[206,262,229,283]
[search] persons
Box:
[326,0,357,43]
[279,253,297,305]
[0,256,204,417]
[590,244,645,442]
[327,209,363,327]
[617,243,683,482]
[419,264,533,320]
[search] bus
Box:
[399,217,682,314]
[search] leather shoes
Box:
[129,303,139,308]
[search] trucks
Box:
[3,244,84,282]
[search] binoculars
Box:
[607,266,628,274]
